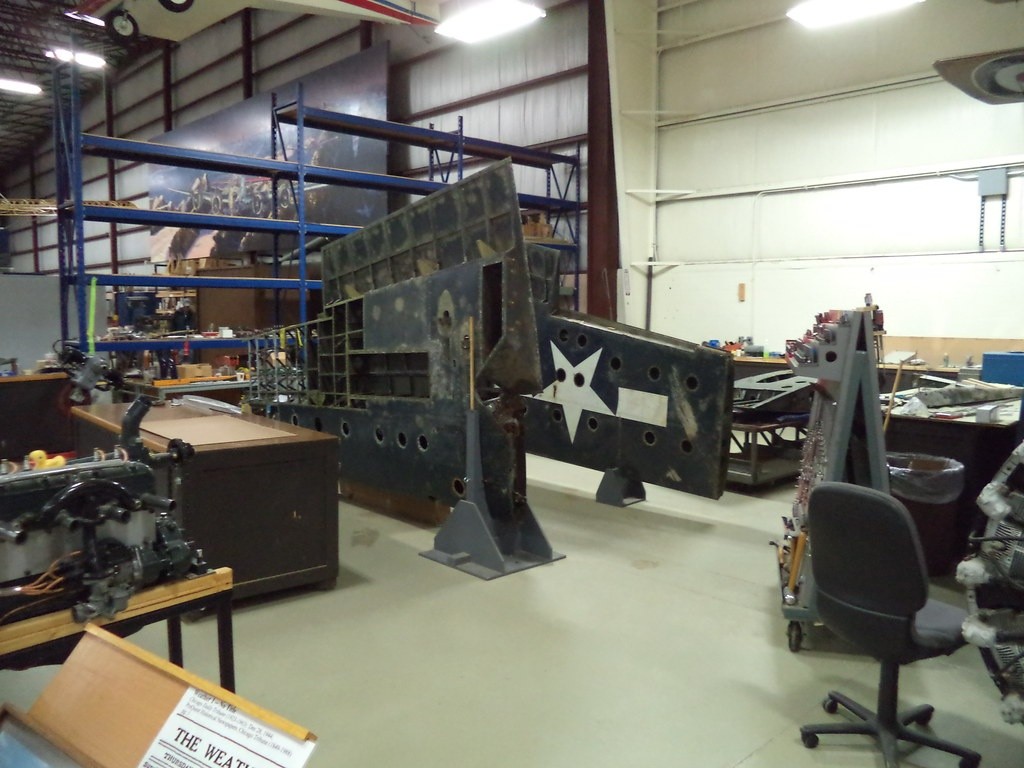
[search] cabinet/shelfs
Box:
[53,107,583,386]
[879,387,1022,563]
[74,399,342,619]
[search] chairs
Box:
[780,483,981,768]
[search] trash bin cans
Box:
[887,453,967,577]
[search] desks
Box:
[0,565,235,701]
